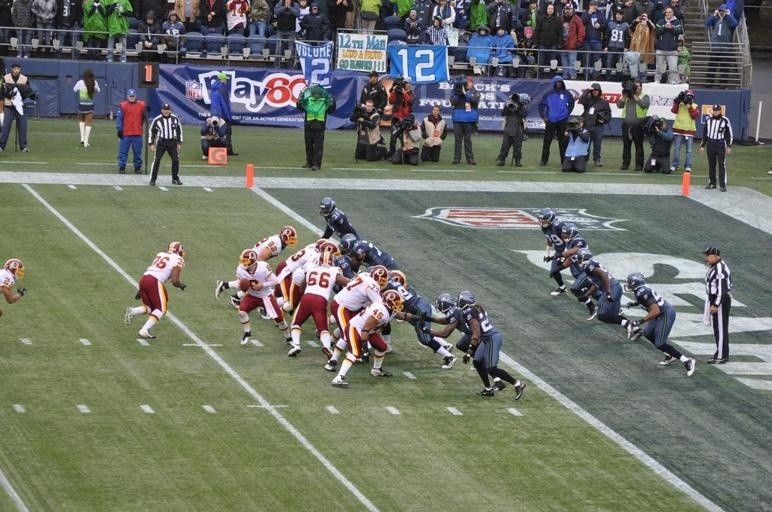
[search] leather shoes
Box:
[496,159,505,166]
[539,160,546,165]
[302,163,310,168]
[468,159,476,165]
[515,159,522,166]
[311,164,320,171]
[452,159,459,164]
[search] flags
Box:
[295,39,333,88]
[387,44,450,85]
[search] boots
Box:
[79,121,86,144]
[83,126,90,149]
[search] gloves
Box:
[421,326,430,334]
[556,253,563,260]
[17,287,28,296]
[145,106,150,112]
[423,315,433,322]
[632,318,645,327]
[607,293,613,303]
[463,349,474,364]
[362,349,370,360]
[579,296,588,303]
[543,253,550,261]
[627,302,637,307]
[118,130,123,138]
[554,265,564,273]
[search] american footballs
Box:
[240,278,250,292]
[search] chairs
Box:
[248,35,267,52]
[23,82,41,121]
[386,28,407,41]
[226,34,246,53]
[269,35,286,53]
[184,32,204,50]
[205,33,225,50]
[381,16,401,28]
[125,17,139,47]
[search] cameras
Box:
[640,115,662,134]
[566,118,581,132]
[622,79,638,97]
[447,74,468,94]
[402,119,412,130]
[355,104,361,114]
[673,90,692,105]
[210,117,218,128]
[389,73,406,93]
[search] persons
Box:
[537,206,580,297]
[431,0,456,29]
[602,9,631,80]
[450,0,470,29]
[492,27,515,77]
[538,76,575,166]
[359,71,388,145]
[0,64,38,153]
[558,3,586,80]
[52,0,82,62]
[531,4,562,68]
[410,0,430,24]
[449,77,480,165]
[389,269,421,298]
[249,0,270,37]
[72,68,101,148]
[331,289,404,386]
[210,73,239,156]
[470,0,488,32]
[644,117,675,174]
[466,26,492,76]
[138,10,161,62]
[545,223,601,320]
[419,106,448,162]
[161,10,186,58]
[174,0,197,32]
[702,247,731,365]
[257,240,328,320]
[627,271,696,377]
[319,243,356,294]
[274,0,300,68]
[297,82,334,171]
[577,84,612,168]
[617,80,651,171]
[299,3,331,39]
[148,103,184,186]
[528,0,684,14]
[133,0,169,23]
[562,116,591,173]
[486,0,513,35]
[288,243,351,361]
[115,89,147,174]
[11,0,36,59]
[456,290,526,400]
[627,12,655,84]
[0,59,5,126]
[404,9,426,44]
[236,249,293,345]
[515,26,536,79]
[125,242,187,340]
[214,225,298,311]
[699,104,734,192]
[324,264,389,372]
[295,0,310,33]
[426,15,450,45]
[318,197,359,240]
[391,115,422,166]
[358,0,383,35]
[350,100,388,163]
[29,0,58,58]
[0,0,12,56]
[581,0,607,82]
[653,6,684,85]
[675,35,692,85]
[388,80,414,157]
[227,0,250,36]
[199,0,227,35]
[81,0,107,62]
[102,0,134,64]
[366,266,457,369]
[669,90,700,173]
[326,0,354,33]
[272,242,341,328]
[200,116,228,160]
[702,3,739,89]
[423,292,479,371]
[493,94,529,167]
[572,247,643,342]
[339,233,399,272]
[0,258,28,317]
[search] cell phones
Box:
[639,17,646,20]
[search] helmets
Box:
[561,223,579,240]
[371,265,387,286]
[389,270,406,288]
[239,250,257,270]
[168,242,184,255]
[321,242,338,255]
[624,273,644,292]
[538,208,555,228]
[5,258,24,279]
[571,247,591,269]
[351,248,365,267]
[319,197,336,216]
[319,253,332,266]
[317,239,327,249]
[280,225,296,246]
[340,235,356,252]
[383,289,402,313]
[456,292,474,309]
[435,293,453,312]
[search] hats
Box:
[127,89,136,96]
[366,70,378,77]
[403,77,411,83]
[466,76,473,82]
[161,104,170,109]
[565,3,573,9]
[434,15,442,21]
[217,73,230,80]
[712,104,721,110]
[702,246,720,256]
[686,89,694,96]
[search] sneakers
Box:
[119,167,125,174]
[631,328,644,342]
[259,308,270,319]
[551,287,567,295]
[685,167,691,172]
[138,329,155,339]
[135,167,142,174]
[445,344,453,353]
[371,368,392,377]
[514,382,525,400]
[324,360,336,371]
[491,382,504,391]
[288,346,301,357]
[229,295,240,309]
[215,280,225,301]
[125,308,133,327]
[670,166,678,171]
[331,375,350,385]
[721,186,726,192]
[660,356,676,365]
[587,307,600,320]
[707,358,725,364]
[287,339,295,348]
[441,355,456,369]
[172,179,182,185]
[150,180,155,186]
[684,358,696,377]
[705,184,716,189]
[241,331,252,346]
[477,388,494,396]
[626,321,631,339]
[322,347,333,360]
